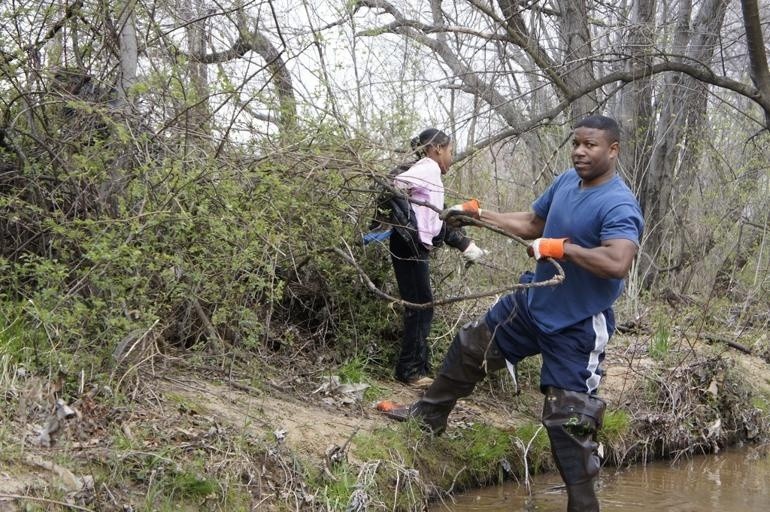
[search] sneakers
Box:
[408,376,436,389]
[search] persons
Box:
[388,128,489,391]
[377,116,646,512]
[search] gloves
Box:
[463,240,484,265]
[527,236,572,261]
[438,199,482,229]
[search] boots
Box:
[542,386,609,511]
[383,316,507,441]
